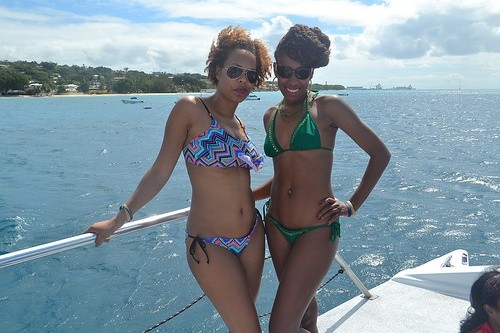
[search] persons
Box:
[250,24,392,333]
[459,267,500,333]
[82,24,274,333]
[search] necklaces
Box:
[278,91,311,120]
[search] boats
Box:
[120,96,145,105]
[245,93,261,101]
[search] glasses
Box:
[223,65,259,84]
[277,65,312,80]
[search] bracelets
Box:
[345,201,356,217]
[119,203,133,222]
